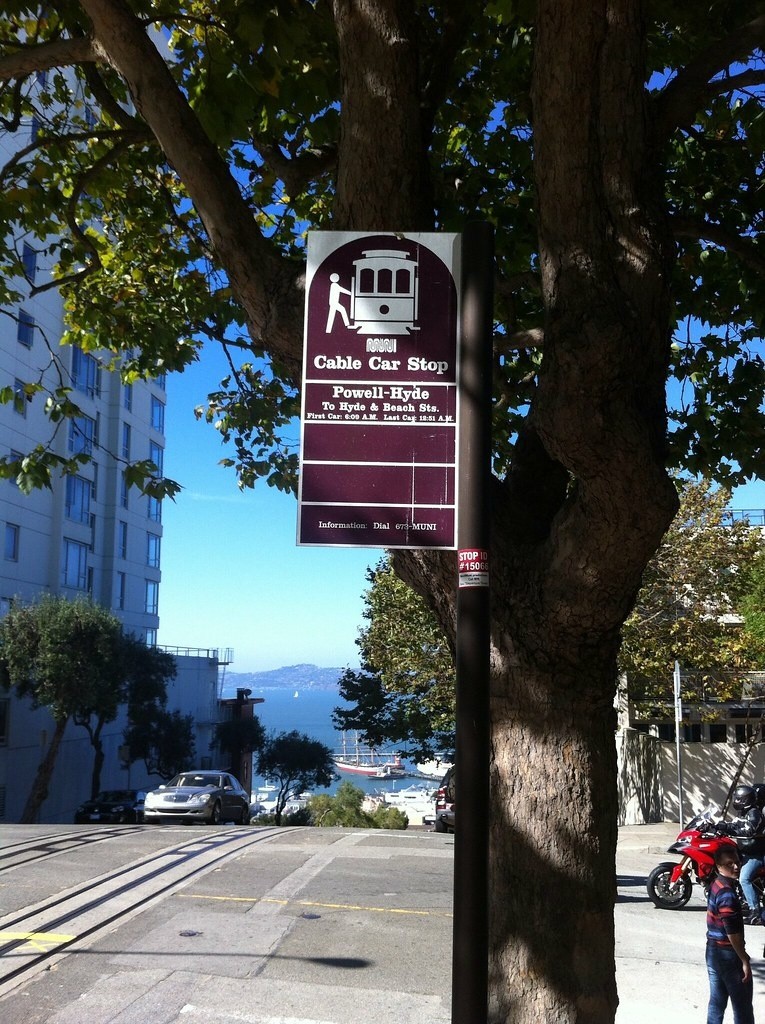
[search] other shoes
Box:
[743,909,761,924]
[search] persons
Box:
[718,786,765,924]
[705,850,754,1024]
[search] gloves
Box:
[715,822,729,834]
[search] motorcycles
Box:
[645,809,765,917]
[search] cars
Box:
[435,762,457,836]
[144,770,250,825]
[75,789,148,825]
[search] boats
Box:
[331,727,408,780]
[249,777,314,817]
[417,753,456,780]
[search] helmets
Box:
[752,783,765,805]
[733,785,756,810]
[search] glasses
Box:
[719,861,741,868]
